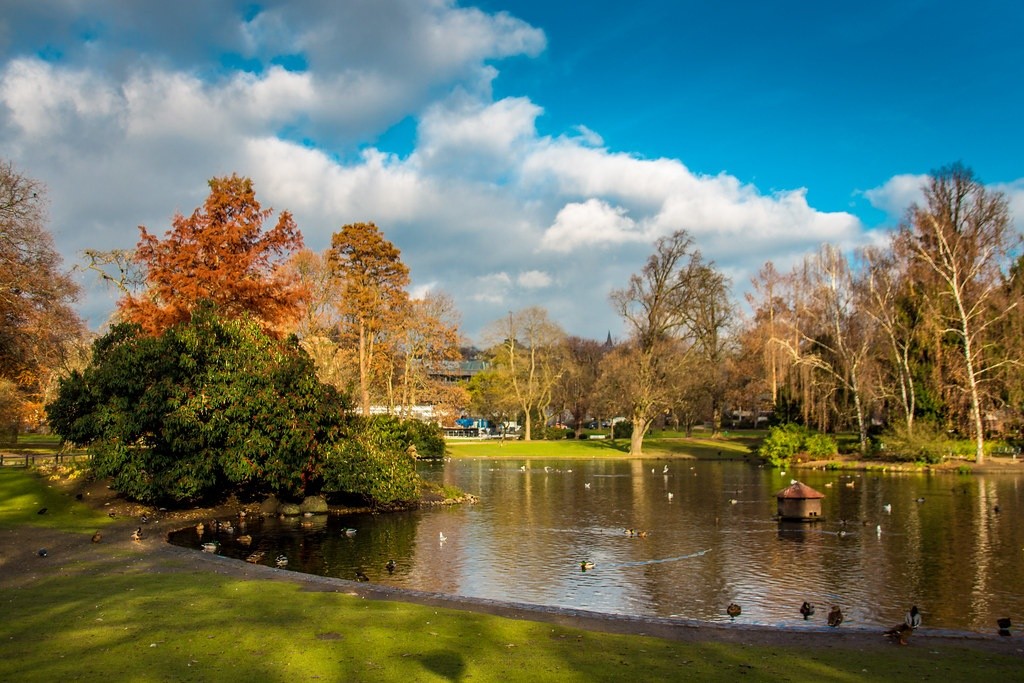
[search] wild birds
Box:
[34,450,1016,642]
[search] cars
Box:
[549,423,567,429]
[589,421,606,429]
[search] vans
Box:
[606,417,626,427]
